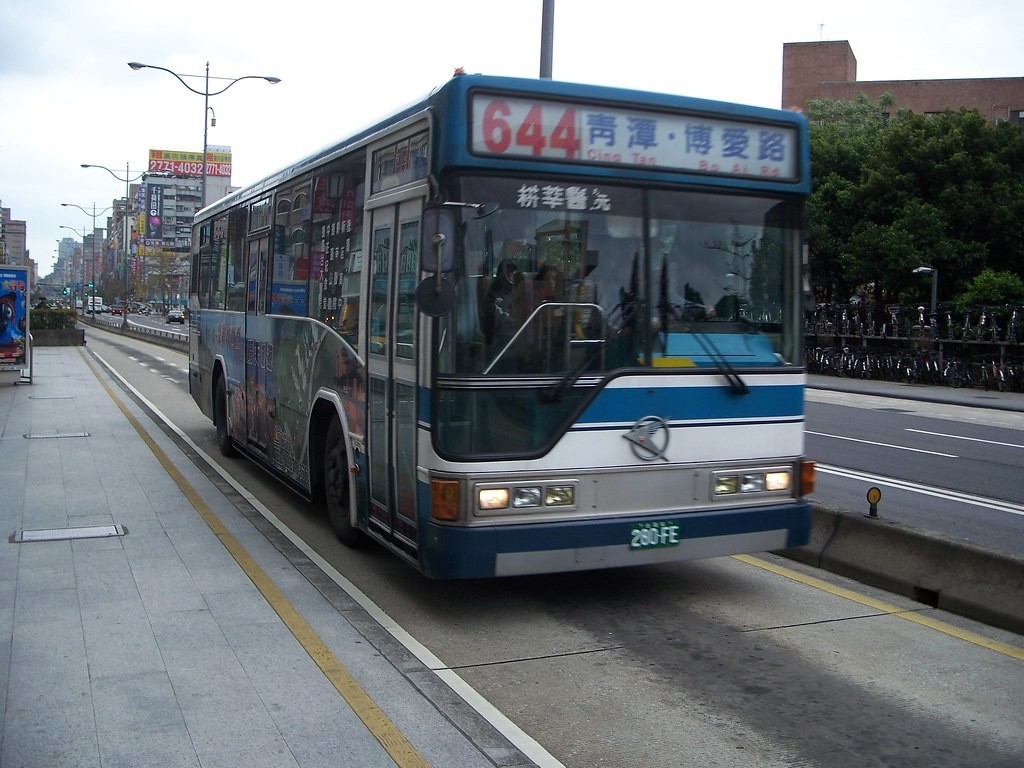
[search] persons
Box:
[657,242,723,317]
[487,259,518,326]
[534,264,561,298]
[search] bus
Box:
[187,66,821,583]
[75,300,83,309]
[86,297,102,314]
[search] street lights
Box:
[913,268,939,314]
[126,62,281,207]
[81,163,182,325]
[49,203,125,322]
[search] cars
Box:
[107,305,123,315]
[166,311,185,324]
[126,305,149,315]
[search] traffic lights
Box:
[88,285,93,287]
[63,291,67,294]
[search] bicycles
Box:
[737,300,1023,392]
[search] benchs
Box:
[466,273,539,361]
